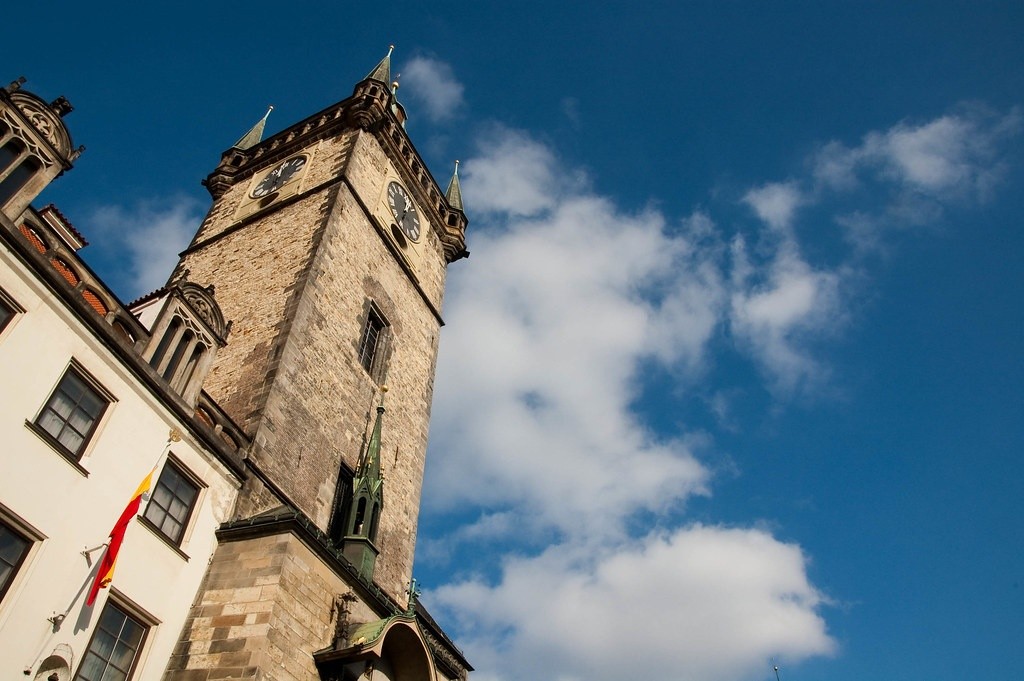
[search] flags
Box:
[86,468,154,606]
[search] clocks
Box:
[387,181,422,243]
[249,152,311,198]
